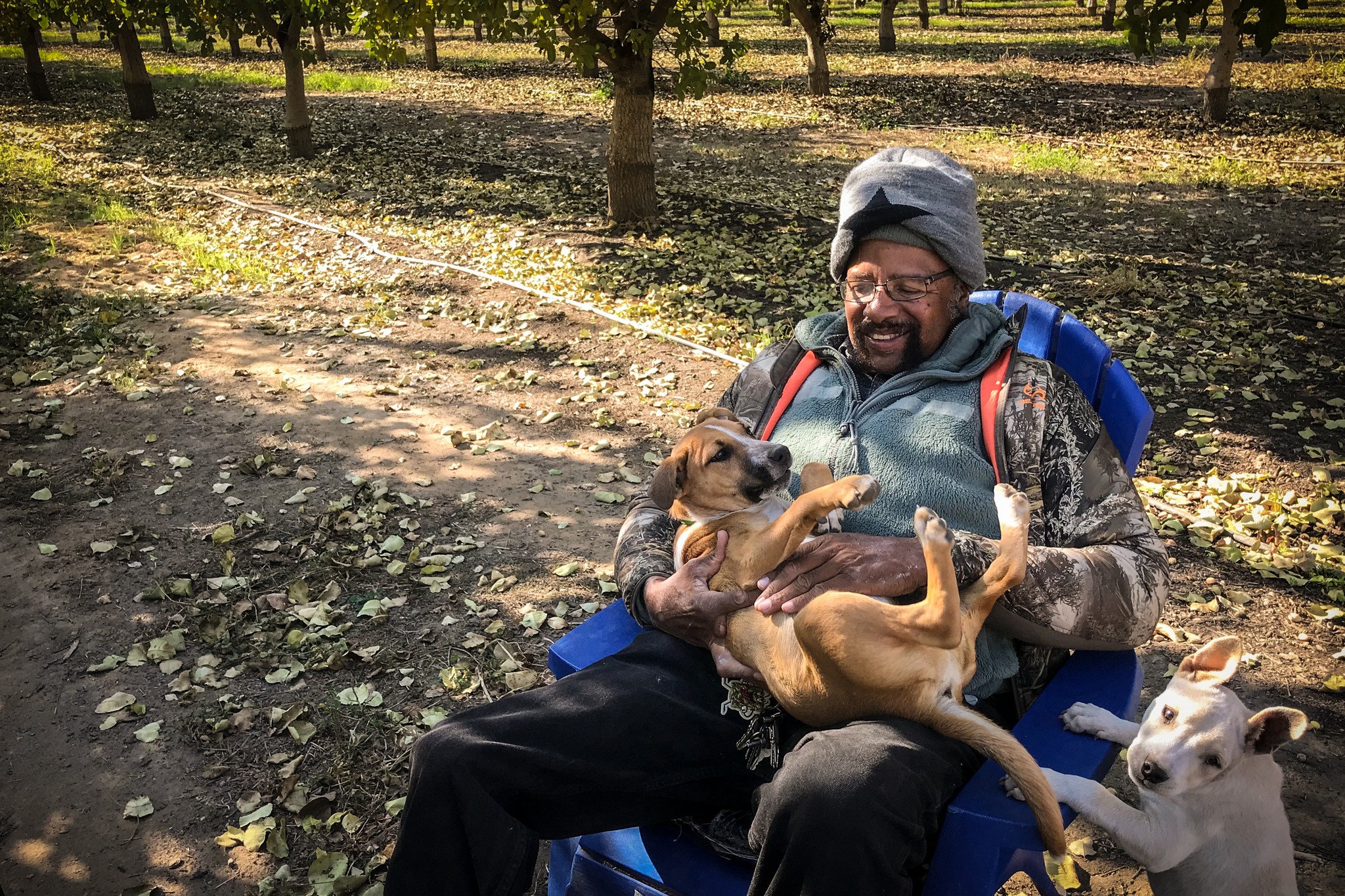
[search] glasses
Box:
[835,268,953,303]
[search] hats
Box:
[831,146,988,288]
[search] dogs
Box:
[1002,638,1309,896]
[650,406,1071,867]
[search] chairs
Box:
[546,291,1155,896]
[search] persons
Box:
[381,147,1170,896]
